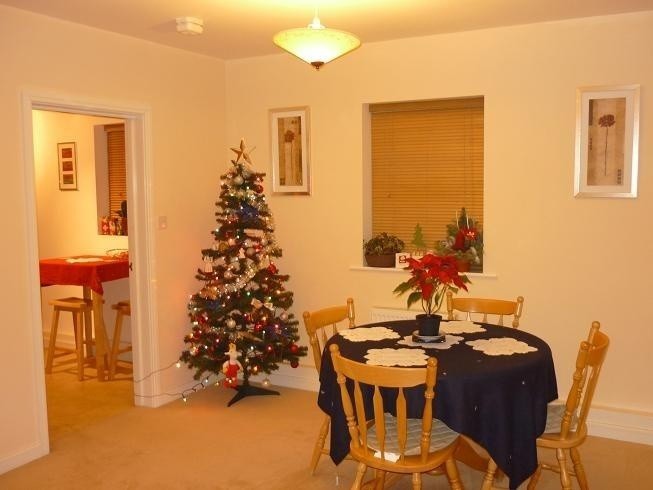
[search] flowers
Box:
[393,254,471,318]
[434,208,483,265]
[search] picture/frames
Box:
[268,105,312,197]
[576,84,642,198]
[57,142,78,190]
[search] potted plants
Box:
[363,233,404,267]
[102,200,128,236]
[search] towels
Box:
[465,338,538,356]
[396,335,464,350]
[335,327,401,342]
[440,321,486,333]
[363,348,430,366]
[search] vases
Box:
[416,314,442,336]
[456,258,471,273]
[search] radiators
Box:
[369,308,456,322]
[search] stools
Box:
[108,300,133,380]
[45,296,104,381]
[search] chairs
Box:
[329,344,466,490]
[446,292,524,328]
[482,322,611,490]
[303,298,358,475]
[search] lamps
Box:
[272,9,363,70]
